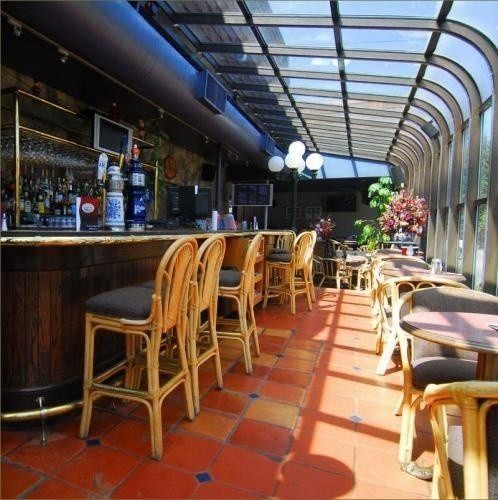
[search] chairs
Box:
[261,232,314,314]
[68,236,200,462]
[360,245,374,253]
[421,380,498,499]
[374,258,429,284]
[134,234,227,414]
[194,235,264,374]
[275,229,296,253]
[394,288,497,465]
[312,237,373,291]
[309,229,316,301]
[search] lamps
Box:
[267,140,325,233]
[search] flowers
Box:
[376,188,428,236]
[313,217,335,236]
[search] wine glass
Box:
[0,136,98,169]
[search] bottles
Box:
[242,220,248,231]
[0,164,98,196]
[212,211,218,231]
[146,183,155,230]
[125,144,146,231]
[0,196,75,232]
[225,200,233,214]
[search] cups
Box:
[430,257,443,275]
[399,247,408,255]
[411,247,418,256]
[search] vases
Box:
[393,232,413,245]
[317,237,322,241]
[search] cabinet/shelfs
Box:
[231,237,264,309]
[0,87,159,239]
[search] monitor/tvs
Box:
[231,183,274,207]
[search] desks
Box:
[374,277,471,376]
[383,267,466,283]
[376,249,402,254]
[399,310,497,382]
